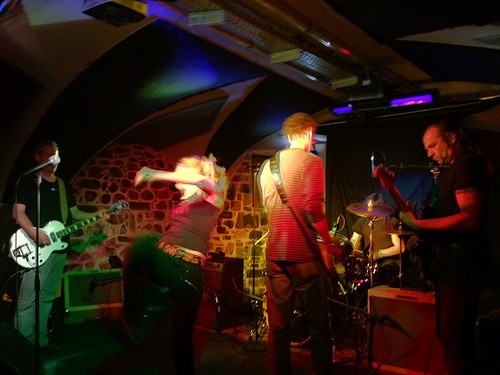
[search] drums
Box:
[343,256,370,280]
[263,281,349,346]
[328,230,355,274]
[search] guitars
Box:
[317,242,340,297]
[9,199,129,269]
[373,163,423,273]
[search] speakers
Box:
[368,285,448,375]
[62,268,126,323]
[193,256,243,329]
[0,319,45,375]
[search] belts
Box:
[158,241,205,266]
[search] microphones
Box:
[23,155,61,175]
[88,276,95,297]
[370,155,375,177]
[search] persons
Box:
[394,117,500,375]
[350,192,406,309]
[257,112,340,375]
[99,154,228,375]
[1,139,71,351]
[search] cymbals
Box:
[346,201,398,217]
[382,229,414,236]
